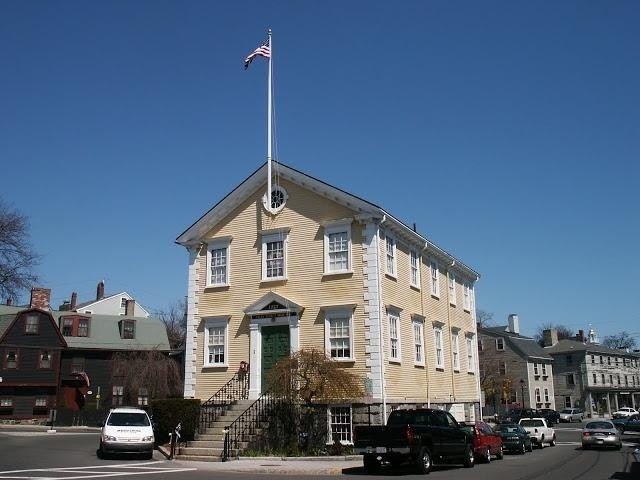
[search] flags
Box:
[244,37,270,71]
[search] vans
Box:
[98,407,157,461]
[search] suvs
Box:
[450,420,505,464]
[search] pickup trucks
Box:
[349,408,479,476]
[518,417,557,450]
[559,408,584,423]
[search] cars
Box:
[612,407,640,420]
[489,423,533,456]
[582,420,623,451]
[610,414,640,435]
[535,409,562,425]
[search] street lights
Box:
[518,378,526,409]
